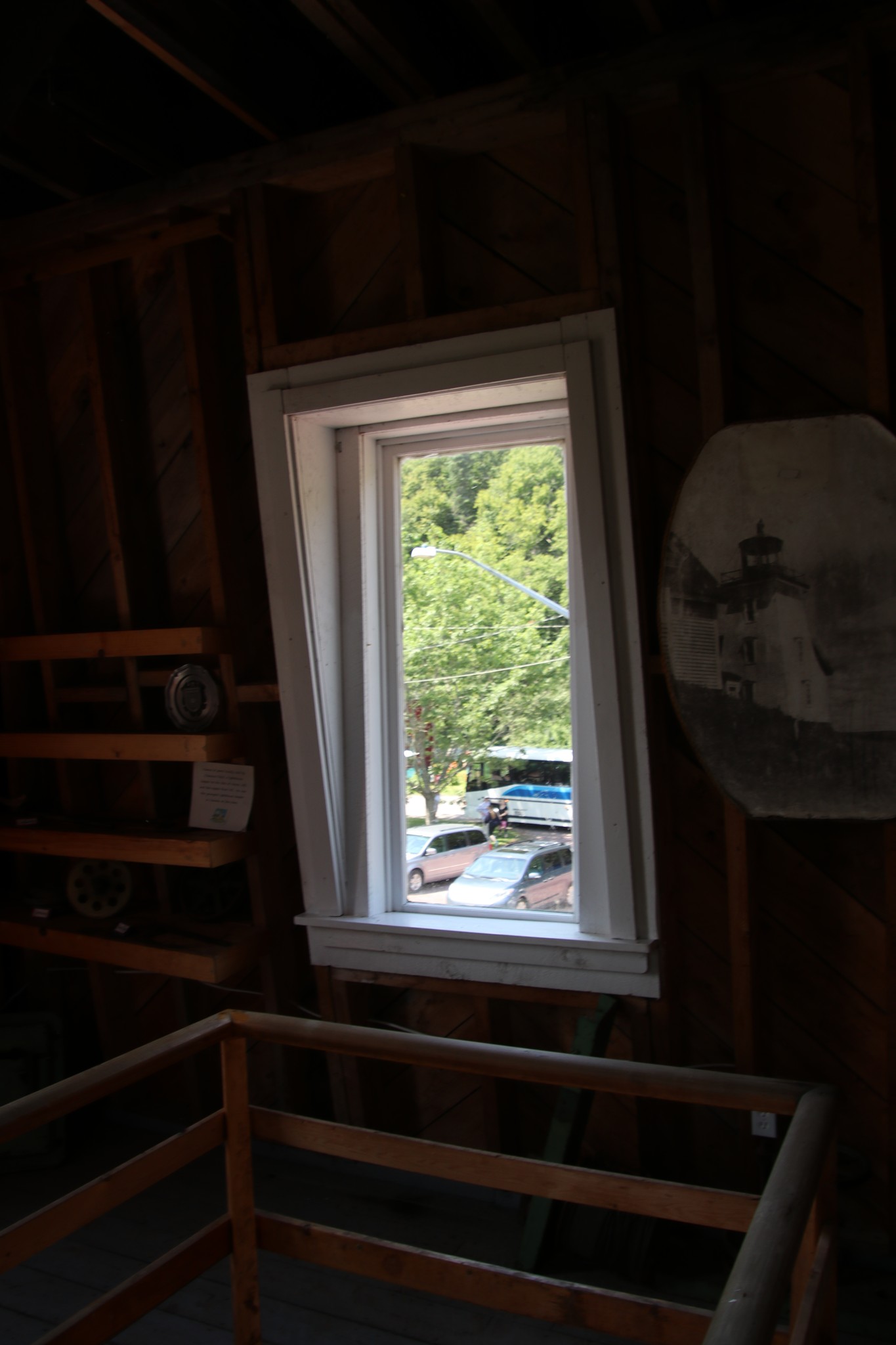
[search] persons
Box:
[476,797,509,836]
[432,791,442,817]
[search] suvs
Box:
[405,822,494,893]
[446,838,574,909]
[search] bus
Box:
[461,745,574,832]
